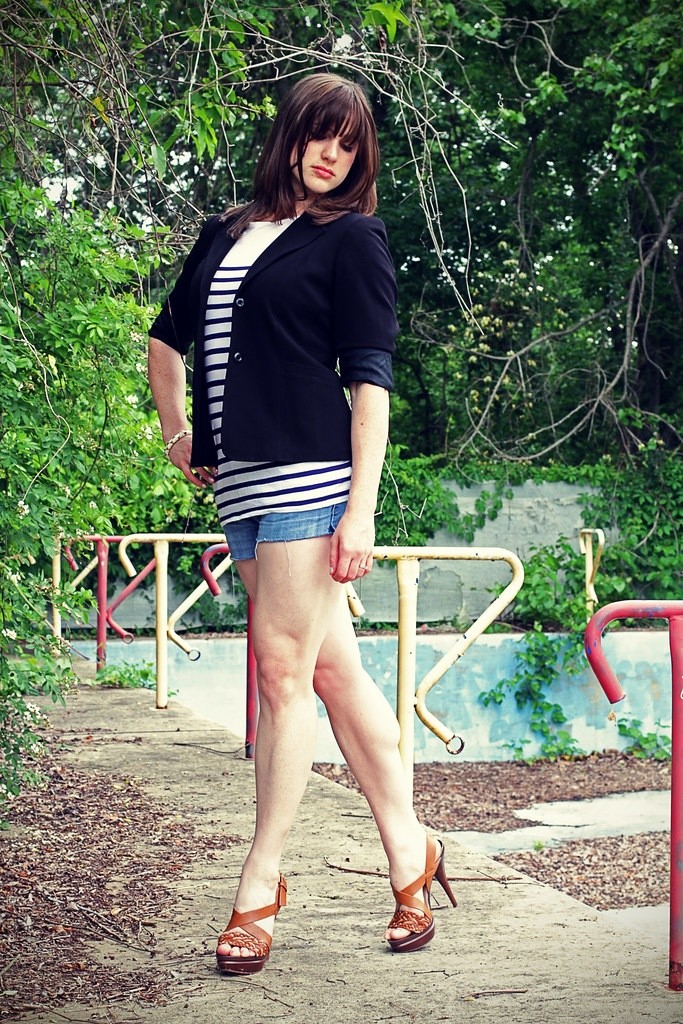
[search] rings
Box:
[359,564,366,569]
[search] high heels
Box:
[384,833,458,953]
[215,871,287,974]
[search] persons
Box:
[149,74,456,975]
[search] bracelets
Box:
[164,430,192,460]
